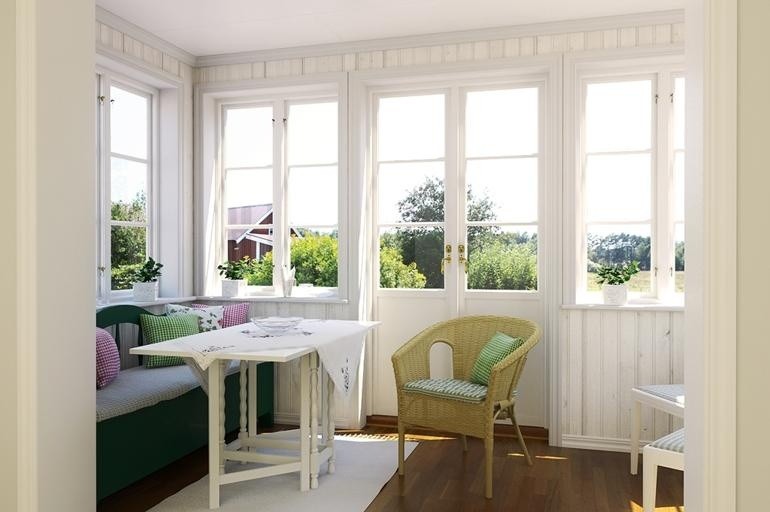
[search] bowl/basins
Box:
[250,315,303,337]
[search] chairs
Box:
[392,315,541,499]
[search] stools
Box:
[631,384,685,474]
[643,428,685,512]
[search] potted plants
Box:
[133,257,163,301]
[597,260,641,302]
[217,261,247,297]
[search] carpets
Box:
[146,429,420,512]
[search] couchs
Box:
[96,305,274,501]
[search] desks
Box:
[129,319,382,508]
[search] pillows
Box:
[140,303,249,368]
[96,327,120,390]
[471,332,525,385]
